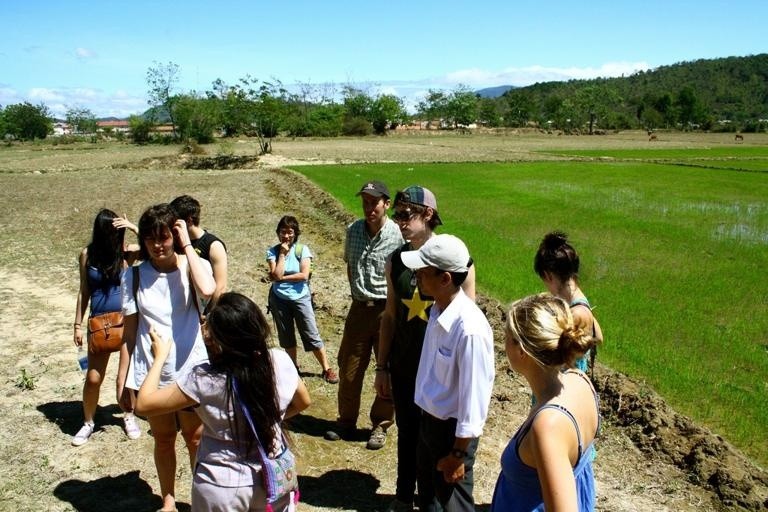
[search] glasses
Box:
[392,210,417,222]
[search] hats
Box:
[401,233,472,273]
[356,180,390,199]
[400,185,444,225]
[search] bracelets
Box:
[74,324,80,328]
[375,364,388,371]
[183,243,192,250]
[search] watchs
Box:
[450,447,469,459]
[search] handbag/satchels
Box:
[231,376,300,512]
[87,312,126,353]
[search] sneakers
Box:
[71,422,95,445]
[123,416,141,440]
[322,369,338,383]
[367,424,387,447]
[325,420,357,440]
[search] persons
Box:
[400,235,494,512]
[170,195,228,363]
[324,181,408,450]
[490,293,600,512]
[72,209,142,446]
[266,215,339,384]
[375,186,476,512]
[532,231,603,407]
[116,203,217,512]
[135,293,311,512]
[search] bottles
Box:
[76,345,89,378]
